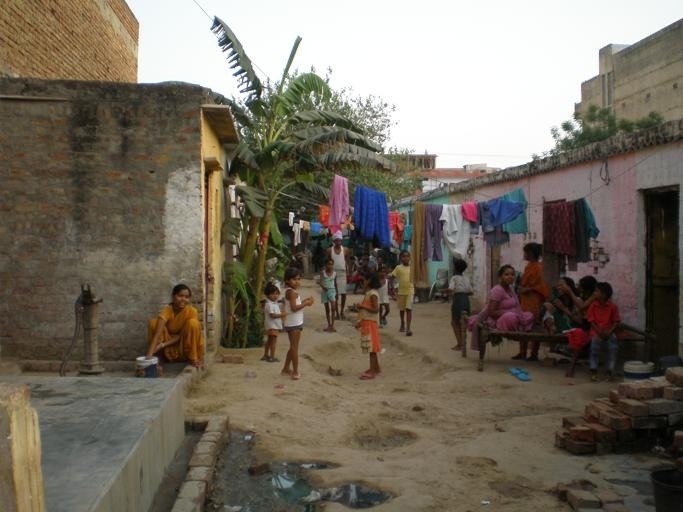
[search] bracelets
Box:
[161,342,165,348]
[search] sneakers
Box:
[517,372,529,381]
[358,370,375,380]
[405,328,414,338]
[267,357,280,362]
[591,372,600,382]
[379,319,383,325]
[399,326,405,333]
[605,373,613,382]
[507,366,529,374]
[382,319,388,325]
[291,371,301,381]
[278,368,292,375]
[260,355,269,361]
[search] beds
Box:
[459,310,659,372]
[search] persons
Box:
[325,230,352,320]
[355,272,384,380]
[320,259,339,332]
[435,257,474,350]
[487,242,621,381]
[349,248,393,293]
[380,268,390,328]
[281,268,314,380]
[147,284,204,366]
[391,250,415,335]
[261,283,288,362]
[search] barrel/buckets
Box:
[135,355,159,379]
[623,360,655,386]
